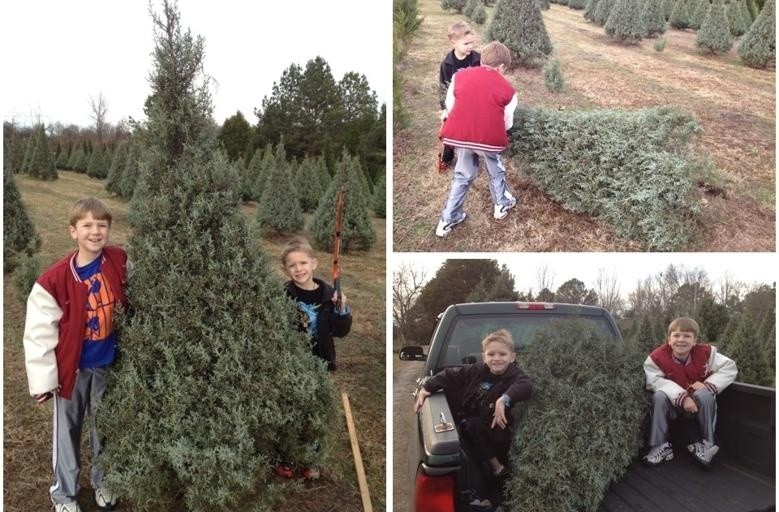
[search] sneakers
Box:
[494,195,517,220]
[277,456,296,476]
[469,464,507,508]
[687,440,720,467]
[642,442,673,465]
[52,501,80,512]
[96,487,117,508]
[303,464,321,480]
[435,212,467,238]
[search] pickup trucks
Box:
[398,299,776,511]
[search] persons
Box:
[274,236,351,481]
[414,329,532,478]
[21,197,135,511]
[641,316,738,465]
[437,42,518,237]
[440,23,479,171]
